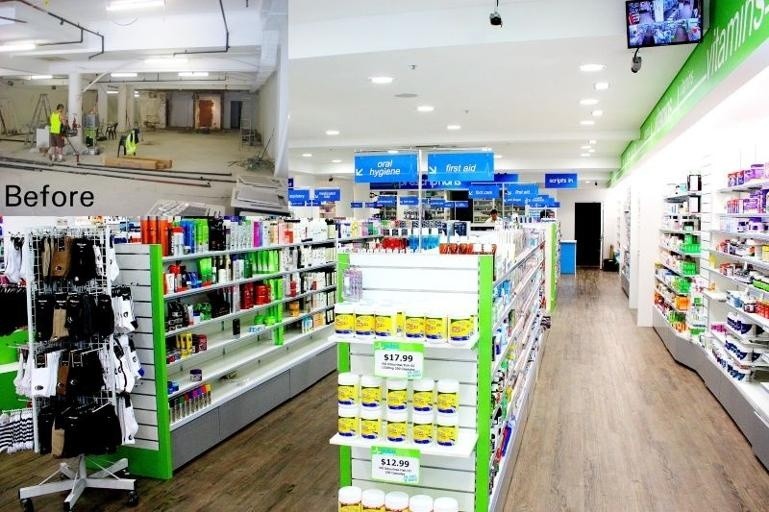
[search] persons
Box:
[47,102,69,161]
[547,210,555,218]
[483,209,503,223]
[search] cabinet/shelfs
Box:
[654,173,703,343]
[702,178,769,428]
[614,191,632,297]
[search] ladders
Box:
[24,94,51,148]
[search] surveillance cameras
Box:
[489,12,502,26]
[631,55,642,74]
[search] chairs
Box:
[105,123,118,141]
[117,128,139,158]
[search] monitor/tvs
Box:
[626,1,704,47]
